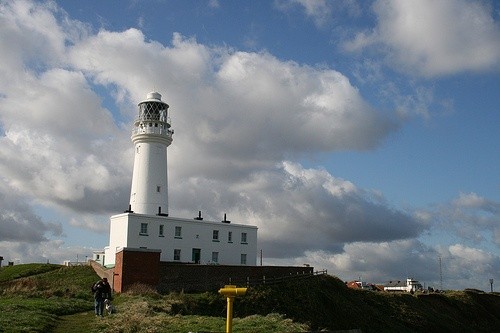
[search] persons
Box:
[102,277,113,308]
[344,279,446,294]
[91,279,106,318]
